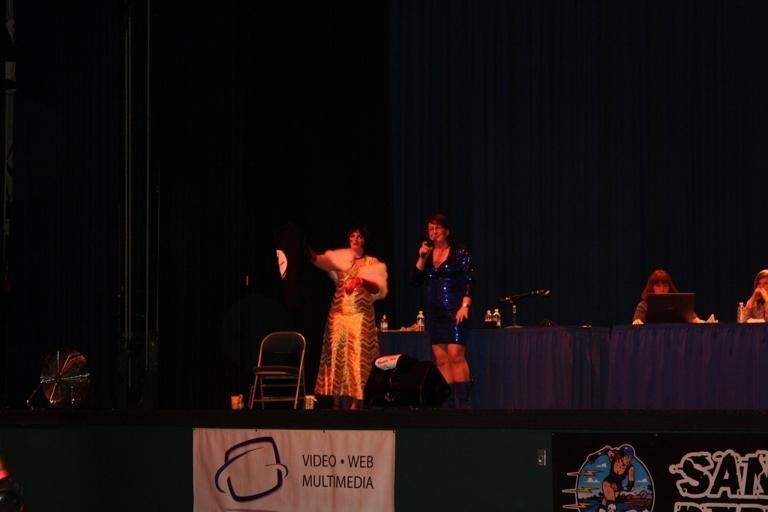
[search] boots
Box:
[442,382,456,408]
[455,382,472,410]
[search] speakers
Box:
[362,358,451,406]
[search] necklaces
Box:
[354,255,365,261]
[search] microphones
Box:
[527,288,552,298]
[422,240,434,257]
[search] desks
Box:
[362,323,768,410]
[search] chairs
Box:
[248,331,307,411]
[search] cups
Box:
[305,395,315,409]
[230,395,240,410]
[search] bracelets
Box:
[359,277,363,286]
[461,304,471,309]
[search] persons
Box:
[745,270,767,322]
[416,213,477,411]
[309,223,388,411]
[634,269,718,325]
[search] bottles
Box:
[416,310,425,331]
[485,307,501,327]
[380,314,387,330]
[737,301,745,323]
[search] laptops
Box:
[645,293,696,322]
